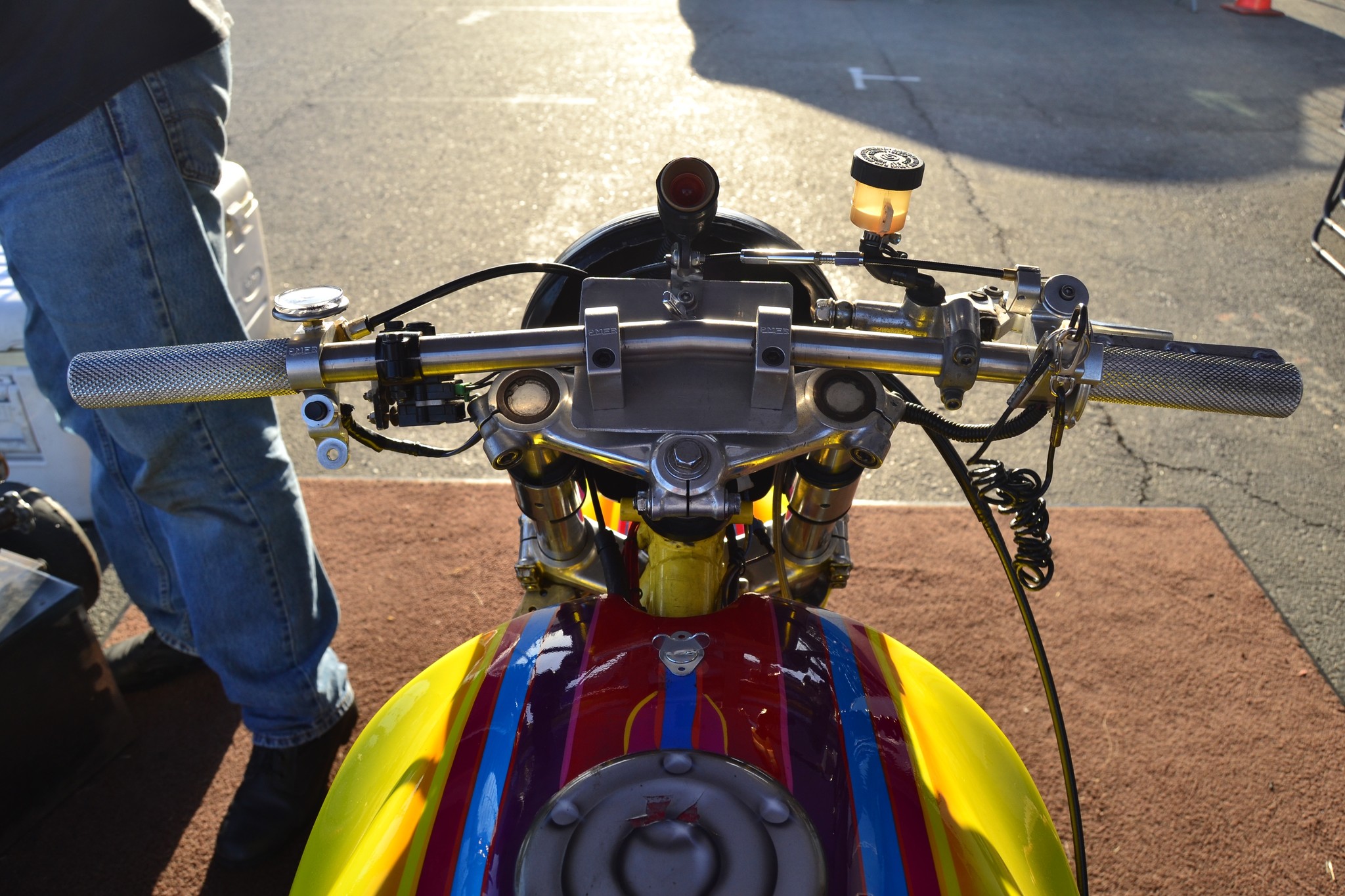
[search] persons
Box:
[0,0,358,875]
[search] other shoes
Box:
[216,703,359,862]
[113,632,205,693]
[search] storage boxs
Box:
[0,163,270,526]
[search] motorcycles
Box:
[69,145,1303,896]
